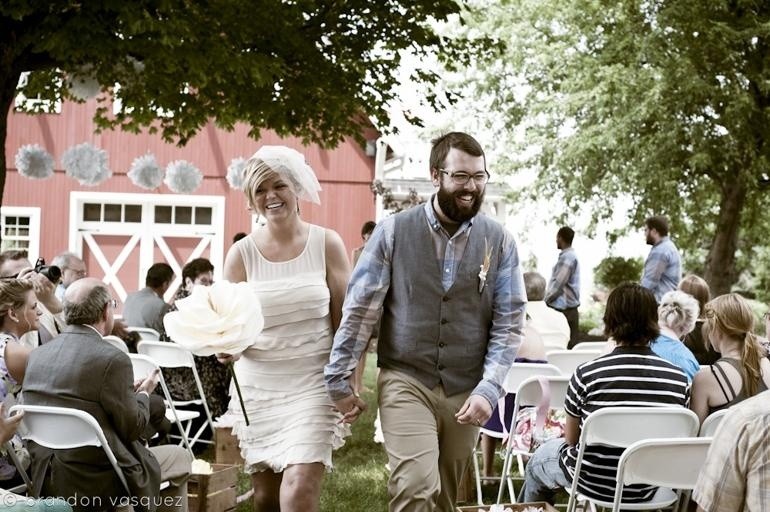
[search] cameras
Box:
[28,258,61,283]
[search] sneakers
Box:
[481,470,498,487]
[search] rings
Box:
[476,419,480,424]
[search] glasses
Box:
[437,165,492,188]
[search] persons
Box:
[233,231,246,242]
[0,249,216,512]
[344,220,376,392]
[208,141,366,512]
[474,270,770,512]
[544,225,581,352]
[321,129,525,511]
[639,215,682,308]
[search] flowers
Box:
[158,276,265,431]
